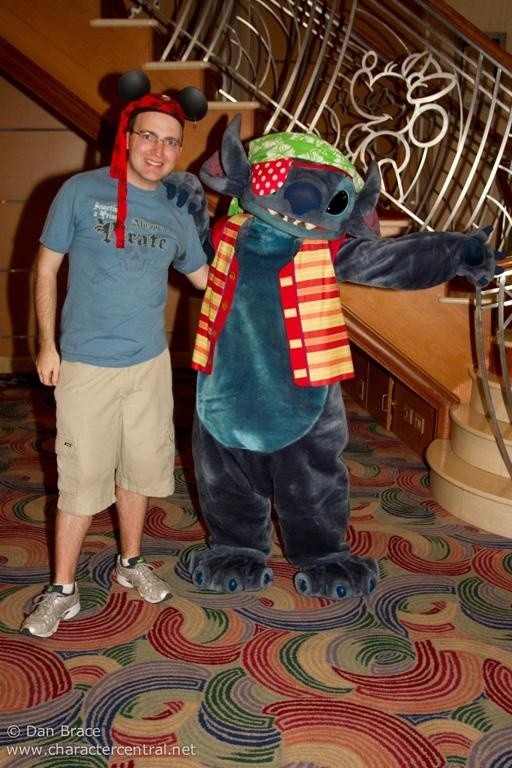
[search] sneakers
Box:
[20,581,83,640]
[113,553,176,605]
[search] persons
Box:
[19,65,214,641]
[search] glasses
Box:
[131,130,182,153]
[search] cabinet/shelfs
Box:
[366,357,395,433]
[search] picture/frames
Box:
[452,30,507,113]
